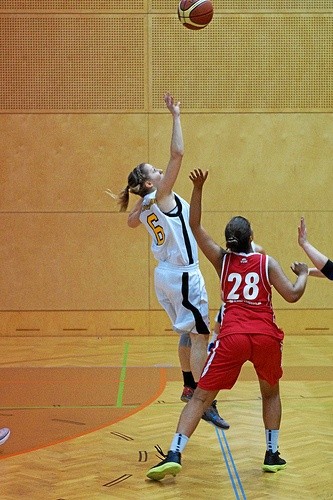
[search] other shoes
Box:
[0,427,11,446]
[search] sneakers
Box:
[202,400,231,430]
[180,386,194,402]
[146,450,182,480]
[263,450,287,473]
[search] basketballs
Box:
[177,0,214,31]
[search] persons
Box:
[146,169,309,481]
[289,216,333,283]
[119,90,230,431]
